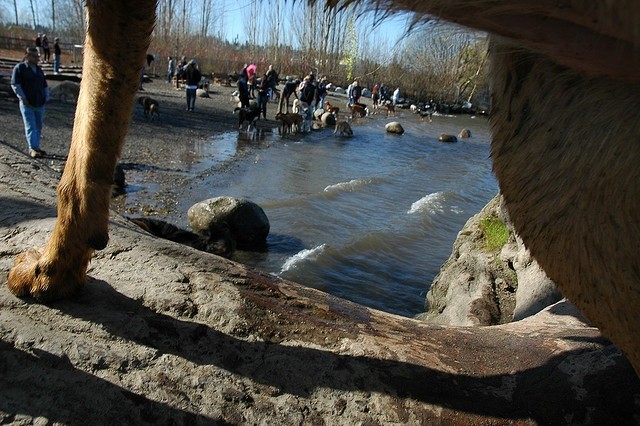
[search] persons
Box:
[35,33,42,61]
[347,81,401,117]
[10,45,50,158]
[185,59,201,113]
[41,34,51,63]
[238,62,333,120]
[140,64,145,92]
[167,55,189,91]
[53,37,62,76]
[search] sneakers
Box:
[30,149,42,157]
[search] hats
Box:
[190,60,196,64]
